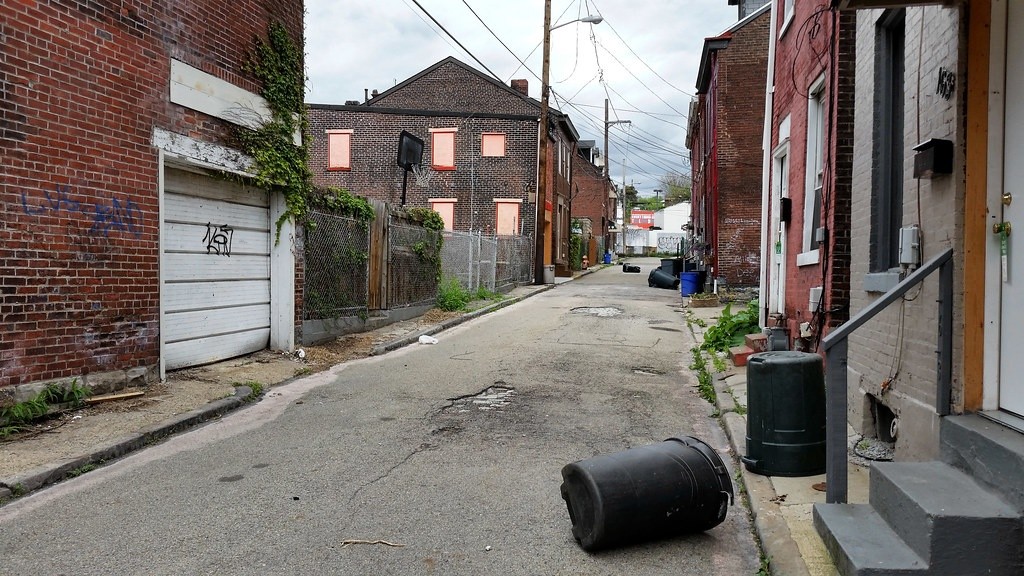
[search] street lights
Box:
[532,14,604,284]
[653,190,663,210]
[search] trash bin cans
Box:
[660,259,677,275]
[679,271,699,298]
[740,353,826,477]
[561,435,734,551]
[603,253,611,264]
[628,265,641,273]
[691,269,707,293]
[648,268,680,289]
[686,262,696,272]
[623,262,630,272]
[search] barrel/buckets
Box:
[741,350,826,476]
[543,265,555,284]
[648,268,680,290]
[689,270,706,294]
[686,263,696,272]
[560,437,734,555]
[680,272,699,296]
[604,254,611,263]
[661,259,674,276]
